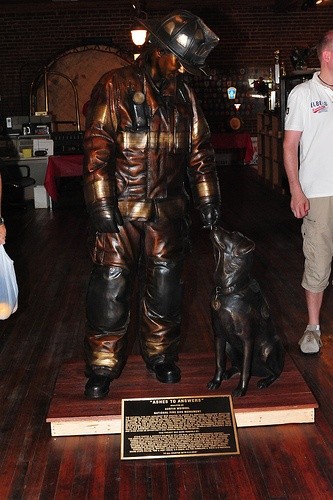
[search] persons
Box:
[0,174,6,247]
[282,29,332,355]
[81,8,223,399]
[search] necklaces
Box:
[318,75,332,87]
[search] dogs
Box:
[208,225,286,398]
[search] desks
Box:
[49,155,84,214]
[211,133,250,167]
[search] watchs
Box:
[0,217,4,223]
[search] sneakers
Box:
[298,325,323,354]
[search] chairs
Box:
[0,159,36,211]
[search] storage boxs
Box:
[216,152,232,166]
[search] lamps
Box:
[130,20,147,49]
